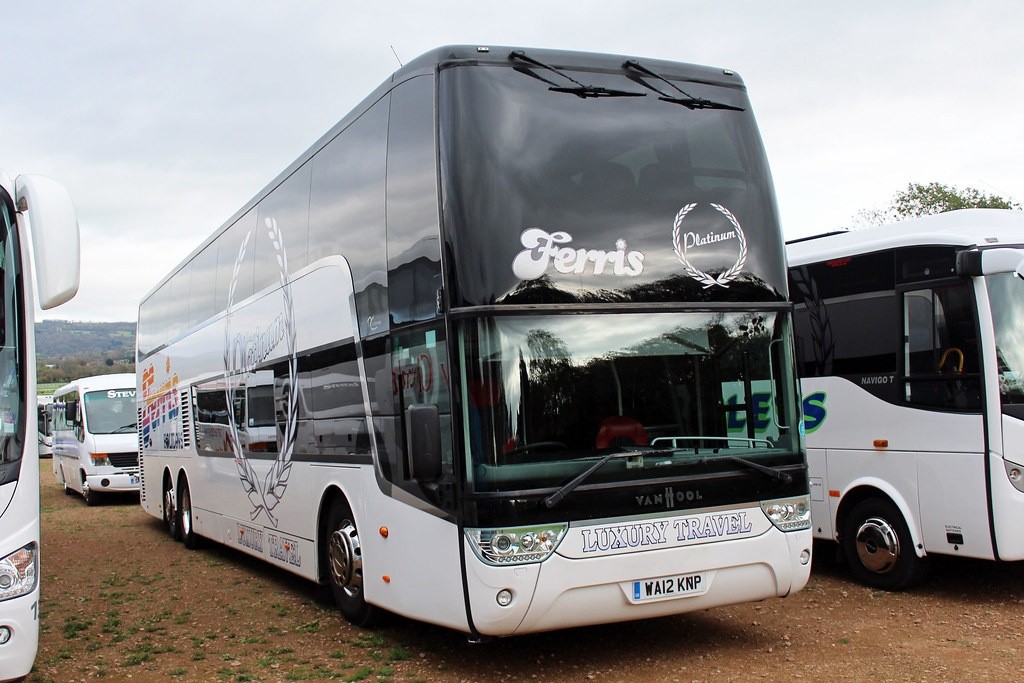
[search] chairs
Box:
[596,413,649,451]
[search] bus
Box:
[131,44,816,644]
[723,205,1024,594]
[35,392,55,458]
[51,371,140,507]
[0,171,81,683]
